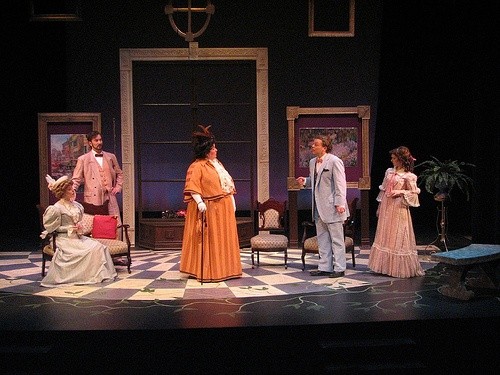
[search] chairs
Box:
[299,196,361,274]
[249,196,292,271]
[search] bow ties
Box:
[95,154,103,157]
[317,159,322,163]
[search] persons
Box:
[67,130,129,267]
[183,138,238,281]
[293,134,348,277]
[368,147,425,276]
[44,179,117,284]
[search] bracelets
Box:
[197,200,203,205]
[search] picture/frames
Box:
[305,0,357,39]
[284,103,371,194]
[26,0,85,24]
[35,111,103,220]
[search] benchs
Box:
[36,202,134,279]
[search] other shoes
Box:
[329,271,343,277]
[310,270,331,276]
